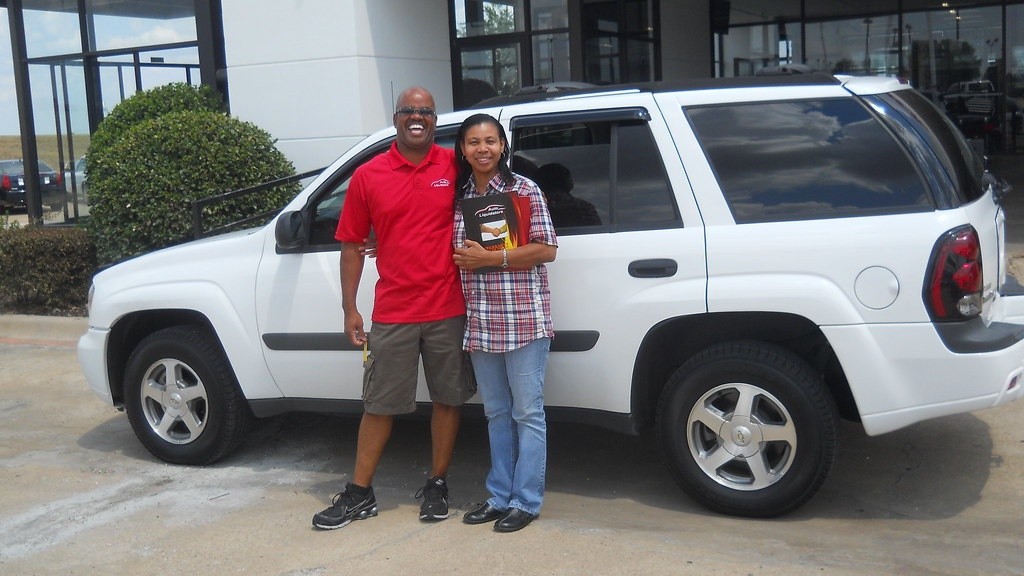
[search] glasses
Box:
[394,107,437,118]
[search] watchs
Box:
[501,248,509,270]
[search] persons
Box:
[358,113,559,533]
[310,86,547,530]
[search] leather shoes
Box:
[494,507,539,531]
[463,502,504,524]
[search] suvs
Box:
[75,68,1023,521]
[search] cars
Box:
[60,153,94,206]
[0,156,63,215]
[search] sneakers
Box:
[415,476,448,520]
[312,486,378,529]
